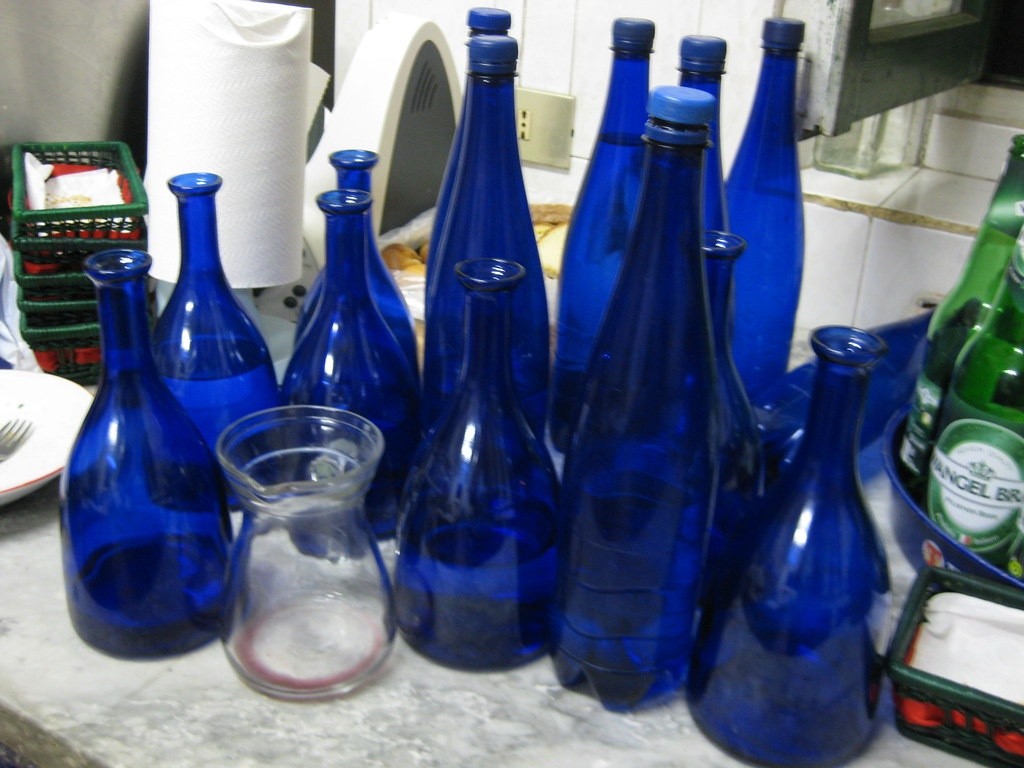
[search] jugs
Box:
[220,406,396,700]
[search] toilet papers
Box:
[142,1,330,291]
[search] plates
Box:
[0,368,96,506]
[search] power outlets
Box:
[512,85,574,169]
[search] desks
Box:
[0,438,992,768]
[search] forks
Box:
[0,421,33,464]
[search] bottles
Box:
[60,247,237,659]
[899,134,1024,580]
[549,86,729,715]
[299,148,419,378]
[423,33,548,441]
[814,0,951,179]
[676,35,728,229]
[395,256,565,676]
[699,227,768,553]
[547,18,656,480]
[749,311,930,503]
[147,171,281,511]
[683,325,890,768]
[425,5,514,289]
[283,191,423,537]
[723,16,807,394]
